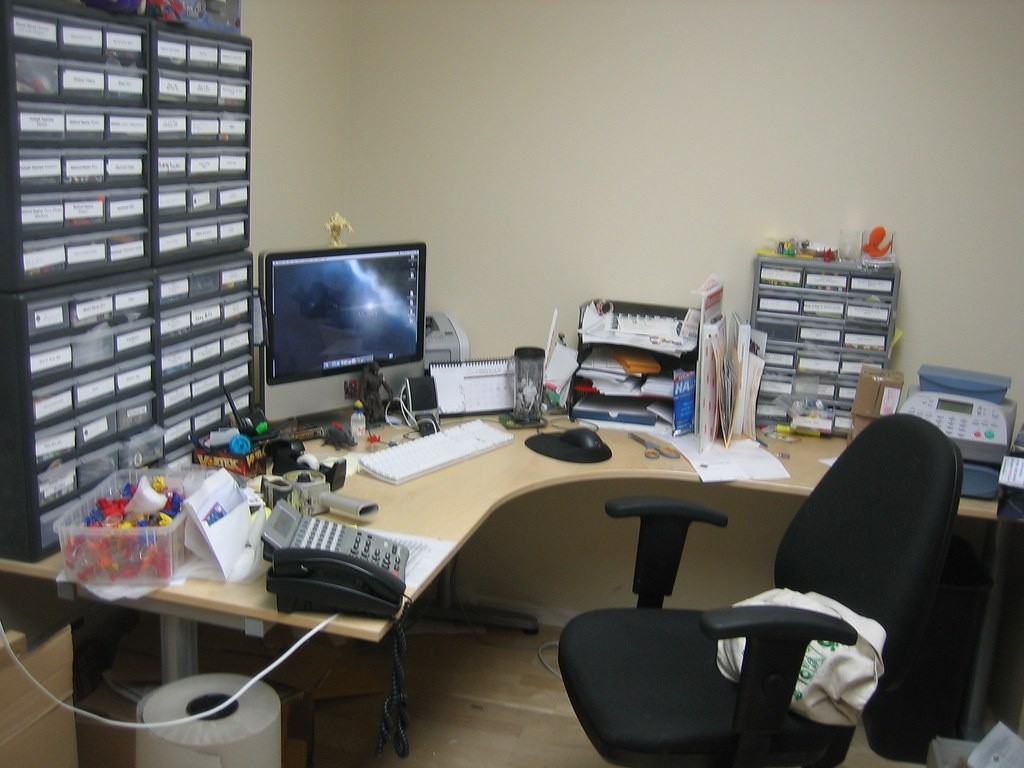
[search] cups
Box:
[513,347,545,422]
[839,229,860,264]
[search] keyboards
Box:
[358,419,516,485]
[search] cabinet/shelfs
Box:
[751,252,901,437]
[566,298,702,423]
[0,0,151,294]
[149,19,252,266]
[156,250,254,466]
[0,269,159,563]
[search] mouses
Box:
[561,428,603,452]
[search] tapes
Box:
[298,454,320,471]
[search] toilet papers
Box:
[131,672,282,763]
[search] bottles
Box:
[350,399,366,447]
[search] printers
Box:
[249,311,471,417]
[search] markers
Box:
[774,423,832,438]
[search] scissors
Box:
[629,432,681,459]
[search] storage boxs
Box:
[840,353,884,375]
[851,365,904,418]
[765,345,794,370]
[843,325,887,352]
[14,4,252,585]
[796,349,839,373]
[846,300,891,323]
[757,400,787,422]
[760,374,791,395]
[845,413,874,449]
[802,295,844,320]
[791,408,834,434]
[795,380,835,401]
[758,290,801,315]
[756,317,798,343]
[837,381,859,402]
[833,410,852,429]
[760,263,804,288]
[805,268,895,295]
[799,322,841,347]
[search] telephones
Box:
[259,500,409,618]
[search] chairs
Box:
[557,413,964,768]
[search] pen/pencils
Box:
[223,386,246,430]
[758,437,782,458]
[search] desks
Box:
[0,412,1024,768]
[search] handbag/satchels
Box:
[716,587,887,727]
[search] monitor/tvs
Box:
[258,242,427,434]
